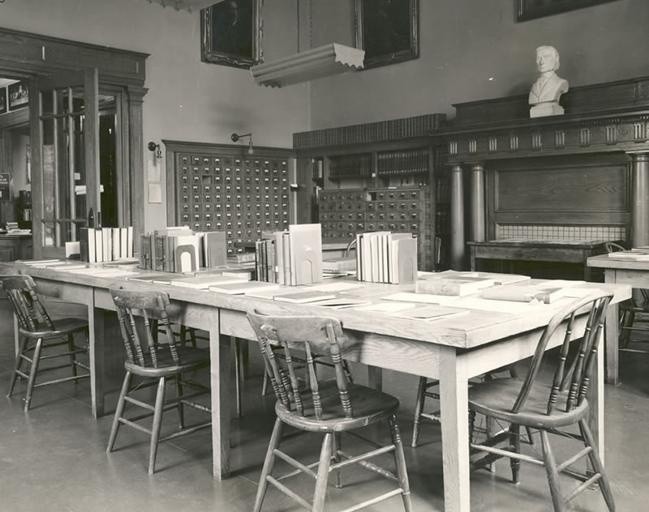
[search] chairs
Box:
[245,308,412,511]
[412,362,536,448]
[468,292,616,512]
[605,242,649,355]
[106,287,210,475]
[0,274,90,411]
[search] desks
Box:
[15,250,631,511]
[466,239,623,283]
[586,247,648,370]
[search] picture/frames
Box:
[511,0,615,23]
[8,80,30,112]
[200,0,264,71]
[0,87,6,114]
[350,0,421,72]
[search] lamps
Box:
[147,141,164,163]
[231,132,256,155]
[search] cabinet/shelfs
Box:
[318,184,436,273]
[162,138,292,260]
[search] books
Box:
[606,243,649,263]
[15,223,580,319]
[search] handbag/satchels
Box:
[605,242,649,307]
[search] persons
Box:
[528,44,569,117]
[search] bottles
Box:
[15,190,32,229]
